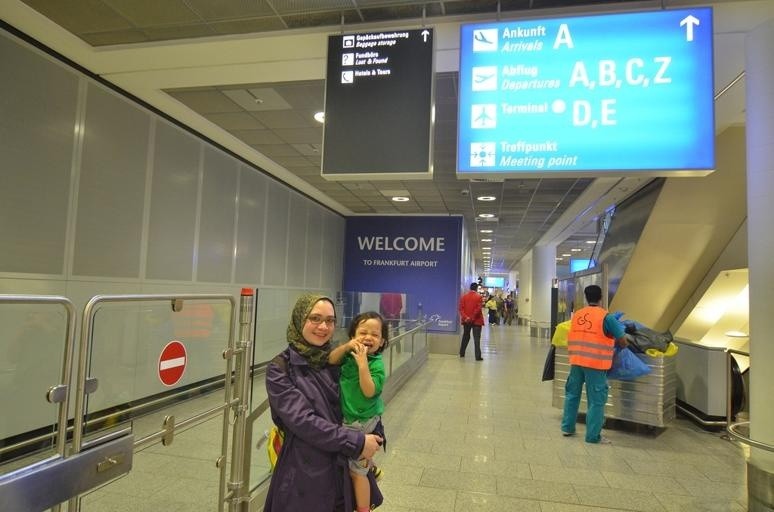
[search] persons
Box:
[264,289,385,512]
[458,282,485,361]
[327,308,388,511]
[378,293,404,355]
[561,285,630,444]
[485,294,515,326]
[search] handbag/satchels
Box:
[267,424,285,468]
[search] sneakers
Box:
[371,465,385,483]
[562,431,579,437]
[476,357,483,361]
[460,352,465,357]
[599,436,612,444]
